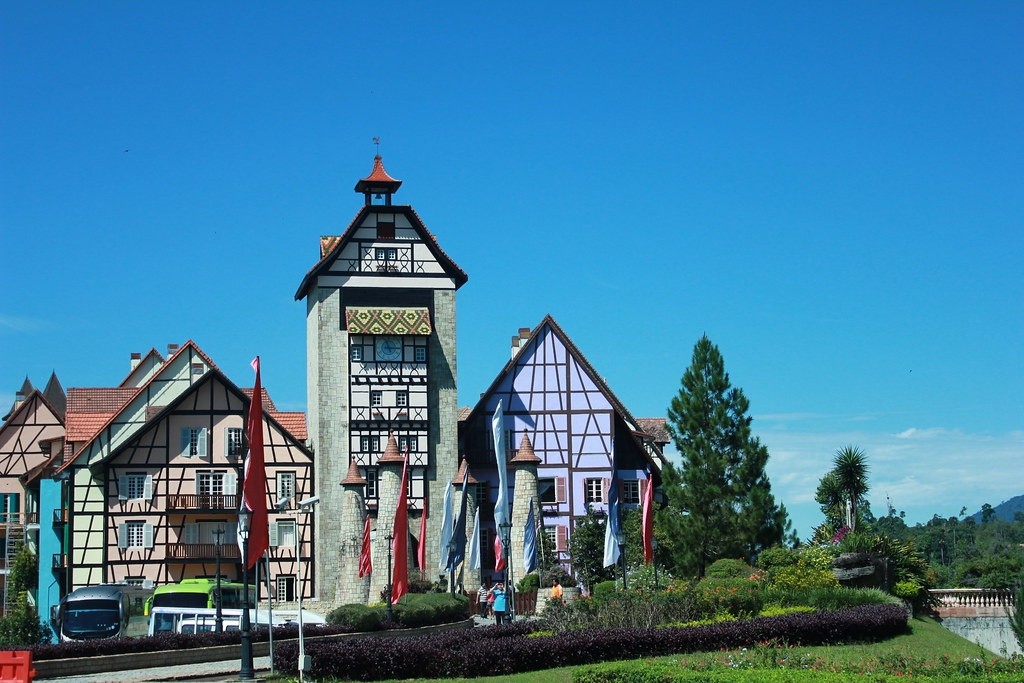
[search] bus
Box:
[54,584,129,645]
[144,578,256,624]
[147,605,288,639]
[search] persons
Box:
[550,578,563,603]
[429,574,518,618]
[579,583,590,598]
[489,581,506,627]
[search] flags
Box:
[467,506,482,572]
[442,462,469,572]
[493,534,508,574]
[235,352,270,575]
[358,513,373,579]
[602,435,626,569]
[522,496,540,575]
[416,496,428,573]
[391,444,410,606]
[489,398,515,559]
[641,473,654,567]
[438,479,454,573]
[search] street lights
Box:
[615,527,628,590]
[578,539,592,596]
[499,517,514,625]
[446,540,457,598]
[384,532,395,617]
[211,523,226,633]
[650,534,659,589]
[233,500,257,678]
